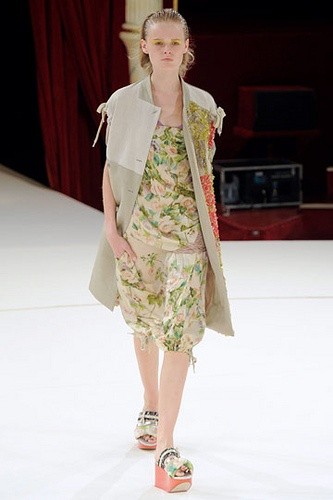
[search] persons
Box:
[87,8,237,495]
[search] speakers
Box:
[212,158,303,209]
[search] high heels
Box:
[134,410,159,449]
[155,448,193,493]
